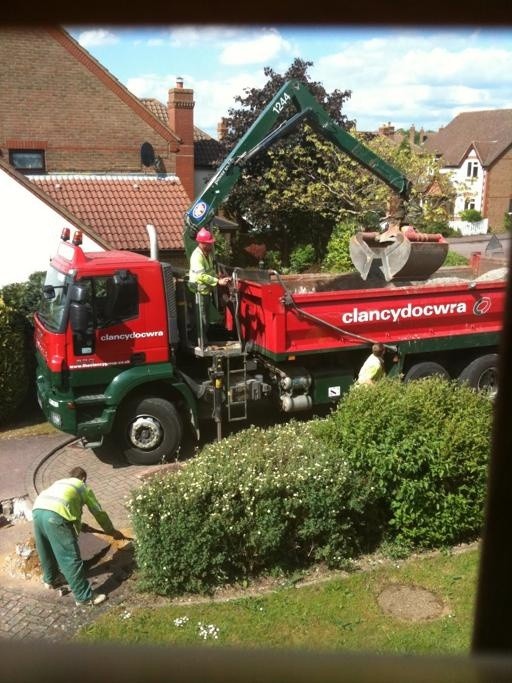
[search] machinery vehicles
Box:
[28,79,511,466]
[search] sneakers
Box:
[75,593,107,606]
[45,577,68,589]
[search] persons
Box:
[30,464,126,607]
[186,227,233,348]
[357,341,388,385]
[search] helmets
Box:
[196,228,215,243]
